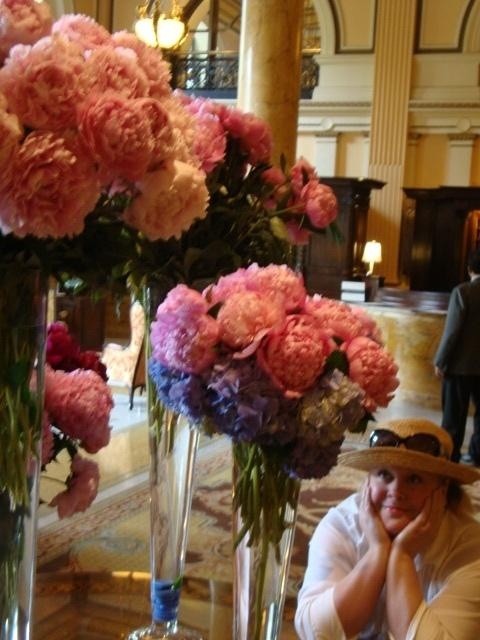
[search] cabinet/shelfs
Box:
[396,185,480,293]
[304,176,387,303]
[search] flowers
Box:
[0,0,212,640]
[133,86,349,456]
[29,323,114,521]
[147,261,398,638]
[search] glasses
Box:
[370,429,440,458]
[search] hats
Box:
[338,418,480,484]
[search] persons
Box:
[293,418,480,639]
[434,255,480,463]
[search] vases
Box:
[130,378,203,640]
[230,435,301,638]
[1,265,48,638]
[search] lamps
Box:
[133,1,188,50]
[361,239,383,277]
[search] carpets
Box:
[364,306,476,418]
[36,418,150,517]
[32,444,479,640]
